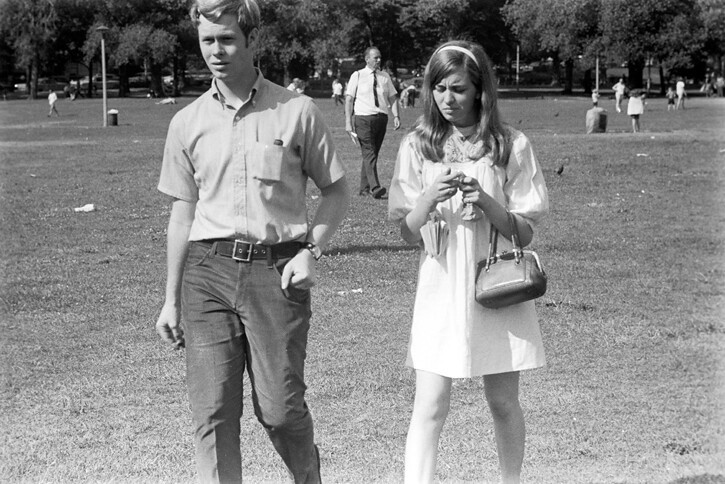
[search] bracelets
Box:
[393,116,400,121]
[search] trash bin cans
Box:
[586,107,608,134]
[108,109,118,125]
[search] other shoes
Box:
[360,187,369,194]
[373,187,386,198]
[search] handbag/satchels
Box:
[475,212,547,308]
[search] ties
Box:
[373,71,379,107]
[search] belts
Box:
[197,240,302,262]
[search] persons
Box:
[47,89,59,117]
[382,65,416,108]
[345,46,401,199]
[286,78,305,95]
[666,87,676,111]
[612,78,626,113]
[700,71,724,99]
[331,78,348,106]
[592,90,601,108]
[386,40,550,484]
[155,0,348,484]
[627,89,645,132]
[676,76,687,111]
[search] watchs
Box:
[300,242,322,260]
